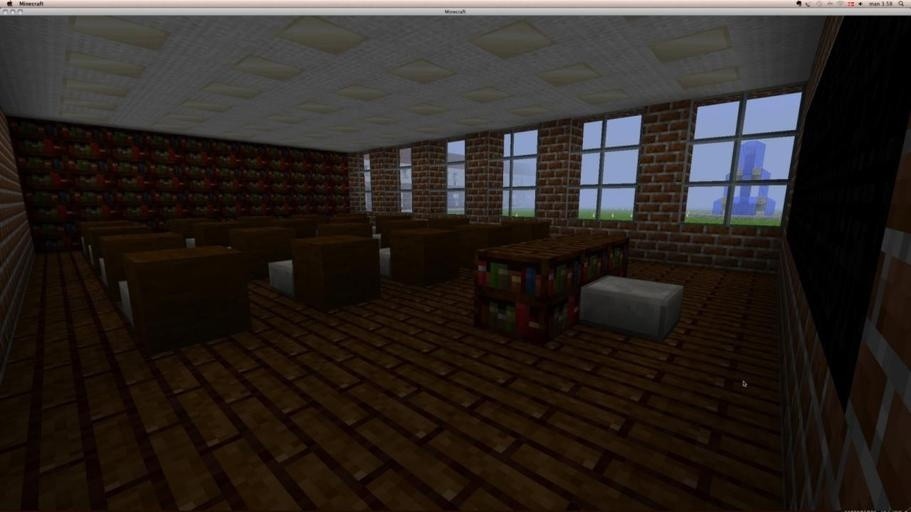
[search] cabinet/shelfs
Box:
[474,235,628,347]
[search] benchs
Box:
[579,274,683,343]
